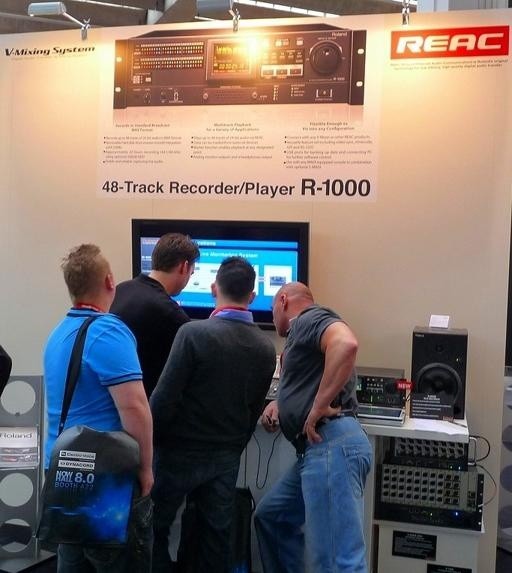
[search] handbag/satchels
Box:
[33,422,143,544]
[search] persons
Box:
[43,244,154,570]
[107,230,200,399]
[252,280,376,572]
[148,257,277,572]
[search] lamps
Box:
[27,1,91,39]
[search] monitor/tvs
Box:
[130,217,310,331]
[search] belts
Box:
[316,409,355,430]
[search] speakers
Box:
[409,327,469,420]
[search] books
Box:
[357,414,407,426]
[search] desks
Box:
[255,413,470,573]
